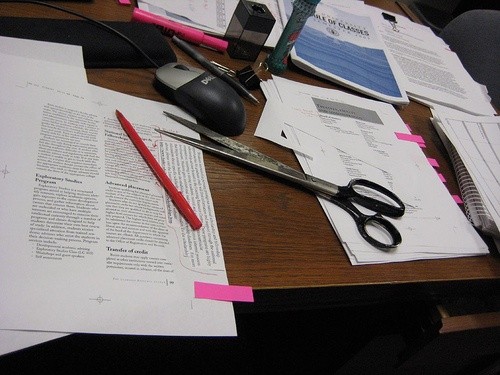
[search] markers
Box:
[131,7,228,52]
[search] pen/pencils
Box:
[116,110,202,231]
[172,36,260,105]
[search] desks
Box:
[0,0,499,375]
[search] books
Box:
[434,103,500,233]
[278,1,409,103]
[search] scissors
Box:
[155,110,405,251]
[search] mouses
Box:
[154,60,247,137]
